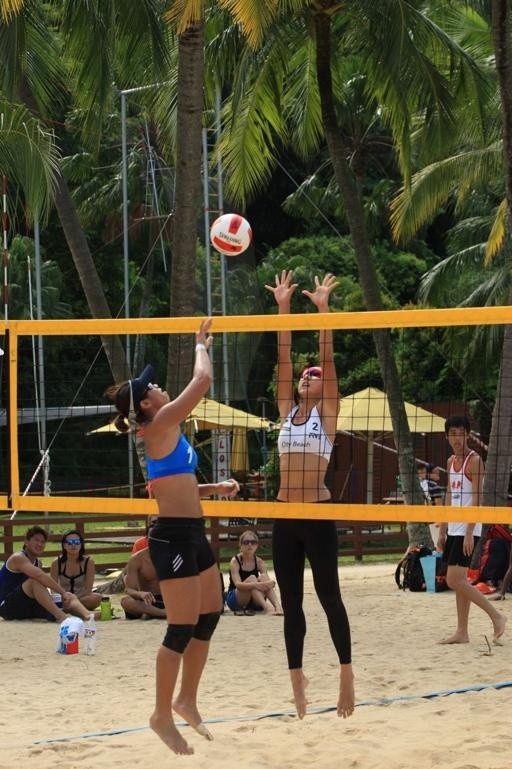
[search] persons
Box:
[51,531,104,610]
[226,530,284,616]
[120,523,167,620]
[419,463,445,528]
[434,415,508,646]
[0,526,114,625]
[130,519,157,556]
[415,463,428,480]
[103,317,241,758]
[264,267,358,721]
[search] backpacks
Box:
[466,523,512,594]
[394,544,433,593]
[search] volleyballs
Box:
[210,211,253,256]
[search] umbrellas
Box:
[83,392,274,446]
[270,385,450,503]
[230,426,250,500]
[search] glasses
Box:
[240,540,259,546]
[63,538,82,546]
[299,368,322,380]
[140,381,154,398]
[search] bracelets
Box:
[193,343,208,354]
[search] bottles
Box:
[50,590,62,610]
[83,614,96,656]
[100,597,112,621]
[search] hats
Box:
[114,363,155,424]
